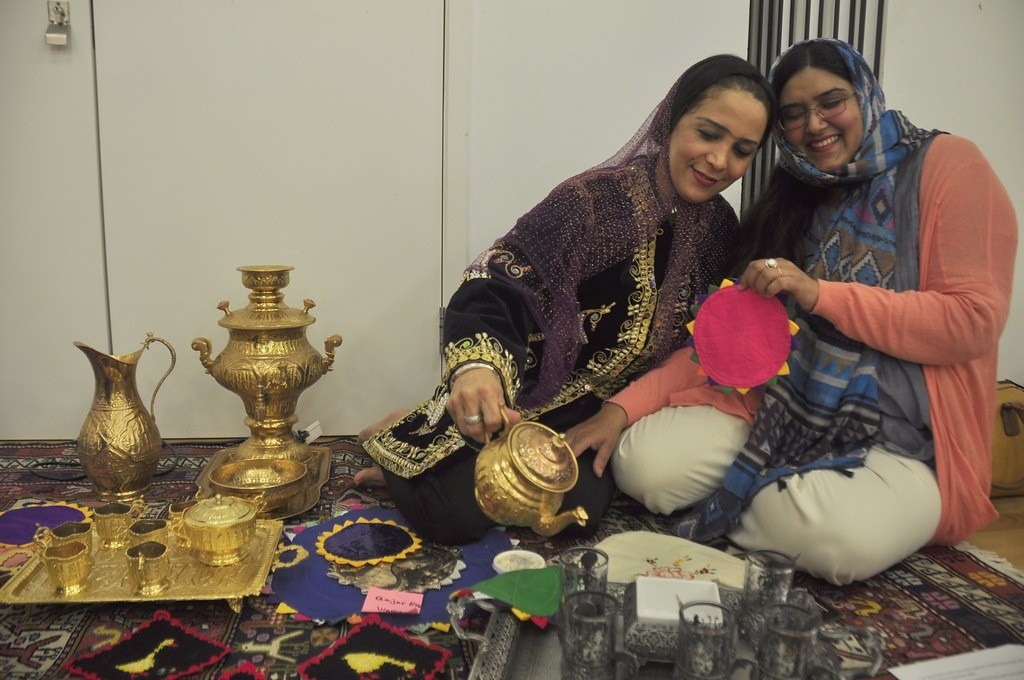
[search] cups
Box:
[185,498,258,564]
[559,547,610,636]
[560,592,617,680]
[679,601,734,680]
[94,503,130,547]
[129,518,169,549]
[127,541,171,596]
[745,547,794,629]
[51,522,94,569]
[170,500,198,537]
[760,604,819,680]
[45,543,88,597]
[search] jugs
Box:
[75,336,177,503]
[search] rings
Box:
[777,268,783,276]
[463,413,481,424]
[765,258,778,269]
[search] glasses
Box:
[776,91,857,132]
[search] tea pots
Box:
[473,404,587,536]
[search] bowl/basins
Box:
[208,460,306,512]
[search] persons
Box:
[356,56,780,548]
[561,37,1017,587]
